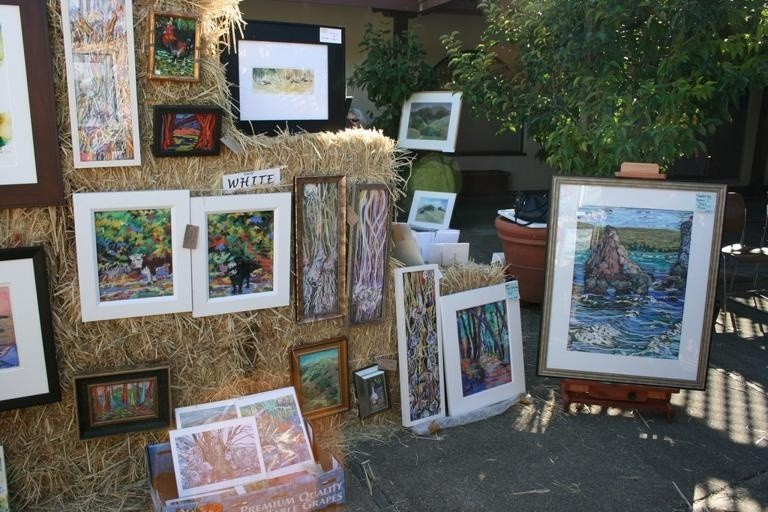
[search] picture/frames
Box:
[396,90,464,153]
[406,189,458,229]
[393,263,446,428]
[536,173,728,391]
[440,279,526,417]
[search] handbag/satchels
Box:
[511,190,550,224]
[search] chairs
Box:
[720,191,768,324]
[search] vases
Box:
[494,215,548,302]
